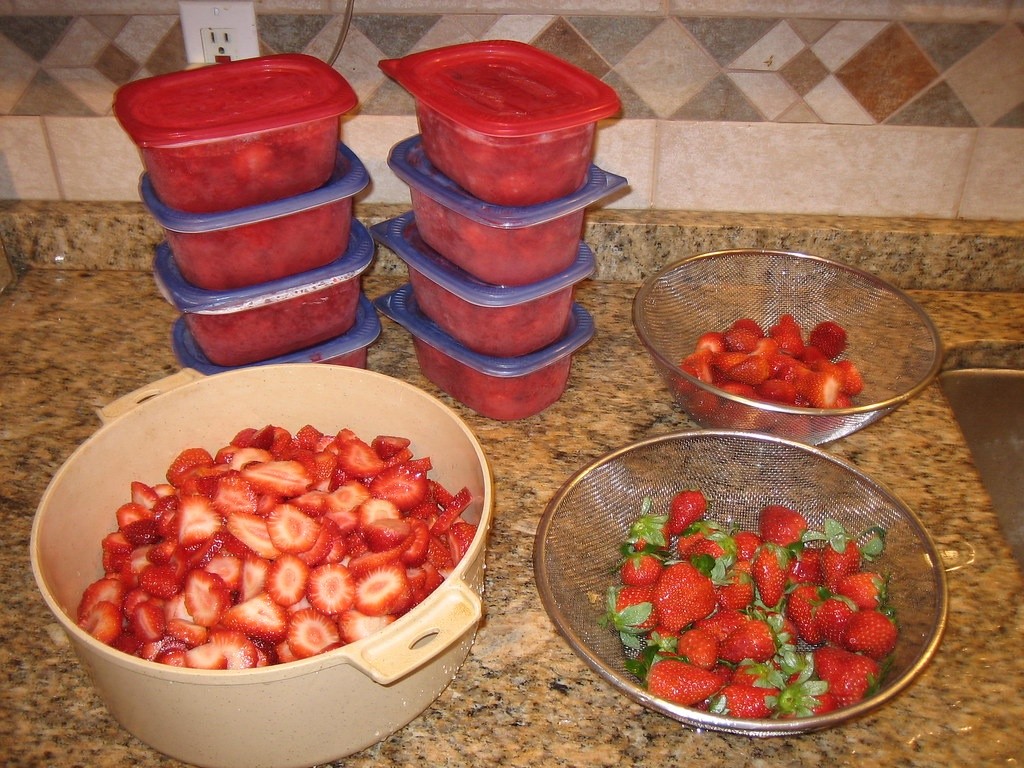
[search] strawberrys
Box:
[673,313,864,442]
[76,424,478,668]
[598,486,901,720]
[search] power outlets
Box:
[178,1,261,64]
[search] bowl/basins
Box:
[150,218,381,376]
[371,211,597,420]
[377,42,621,207]
[28,362,494,768]
[116,51,357,214]
[388,134,627,287]
[136,144,371,291]
